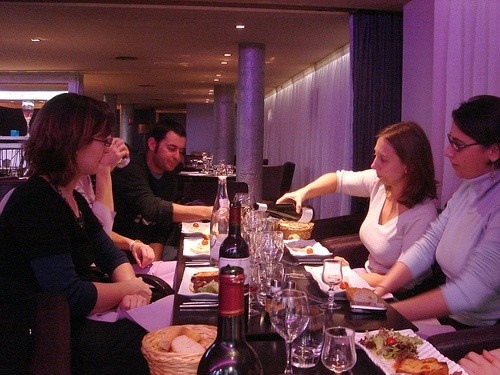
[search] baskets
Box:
[278,220,315,241]
[141,324,218,375]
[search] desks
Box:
[180,171,248,206]
[172,220,420,375]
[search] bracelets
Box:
[375,284,388,292]
[129,239,143,251]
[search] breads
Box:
[346,287,377,307]
[156,325,213,354]
[395,357,449,375]
[191,271,219,294]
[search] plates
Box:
[182,236,210,260]
[283,239,333,259]
[304,265,374,295]
[177,266,249,300]
[344,328,469,375]
[180,221,211,236]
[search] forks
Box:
[281,258,323,267]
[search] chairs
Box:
[191,151,212,161]
[427,323,500,365]
[185,154,203,168]
[31,292,71,375]
[321,232,371,270]
[263,161,296,202]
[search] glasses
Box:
[447,133,483,152]
[91,137,113,147]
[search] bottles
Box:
[218,201,251,325]
[210,176,231,265]
[196,264,264,375]
[254,202,314,223]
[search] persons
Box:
[458,348,500,375]
[93,121,214,265]
[276,121,439,304]
[373,94,500,340]
[0,94,177,375]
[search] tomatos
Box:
[193,223,199,227]
[202,240,208,245]
[307,248,313,254]
[340,281,349,289]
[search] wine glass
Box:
[21,99,34,137]
[321,326,357,375]
[233,193,310,375]
[320,259,343,310]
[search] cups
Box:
[10,166,18,177]
[192,152,234,176]
[105,141,130,168]
[290,305,325,368]
